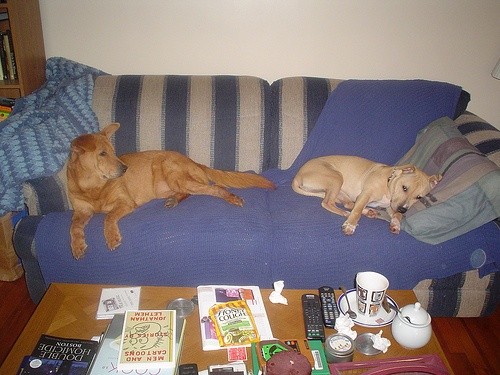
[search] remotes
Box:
[302,294,325,343]
[318,285,339,329]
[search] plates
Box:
[337,289,400,328]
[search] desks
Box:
[0,283,453,375]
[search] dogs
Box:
[292,155,443,236]
[66,122,278,262]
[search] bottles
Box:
[391,302,432,350]
[324,333,355,364]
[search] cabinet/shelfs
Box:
[0,0,47,122]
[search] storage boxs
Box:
[0,211,24,281]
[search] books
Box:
[17,310,187,375]
[251,339,330,375]
[0,31,17,81]
[0,98,16,122]
[197,285,273,351]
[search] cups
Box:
[356,271,390,318]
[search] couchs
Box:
[11,74,500,317]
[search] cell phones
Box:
[179,363,198,375]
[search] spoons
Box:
[340,285,357,319]
